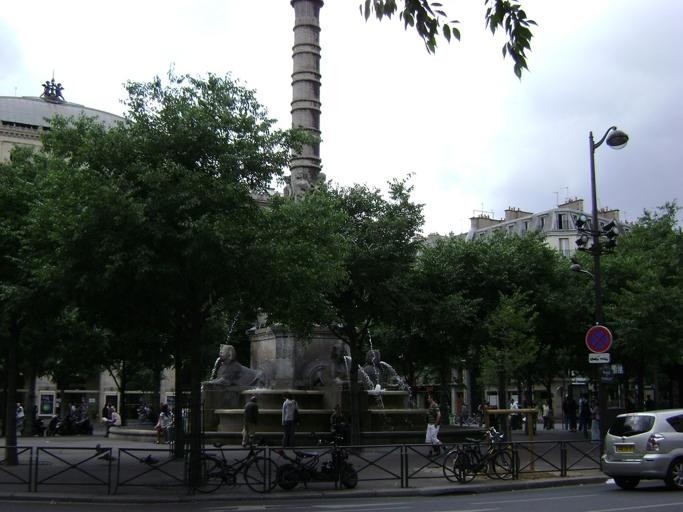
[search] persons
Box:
[152,404,174,445]
[478,403,487,427]
[240,395,260,450]
[279,392,301,448]
[15,402,24,418]
[103,409,122,437]
[424,392,447,457]
[561,390,602,445]
[644,394,656,410]
[107,403,113,417]
[509,398,522,430]
[101,403,108,416]
[329,402,342,431]
[542,399,554,430]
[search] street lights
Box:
[570,125,628,431]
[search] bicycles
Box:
[443,426,520,483]
[187,433,280,493]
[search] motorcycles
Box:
[275,429,357,490]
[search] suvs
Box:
[601,408,682,489]
